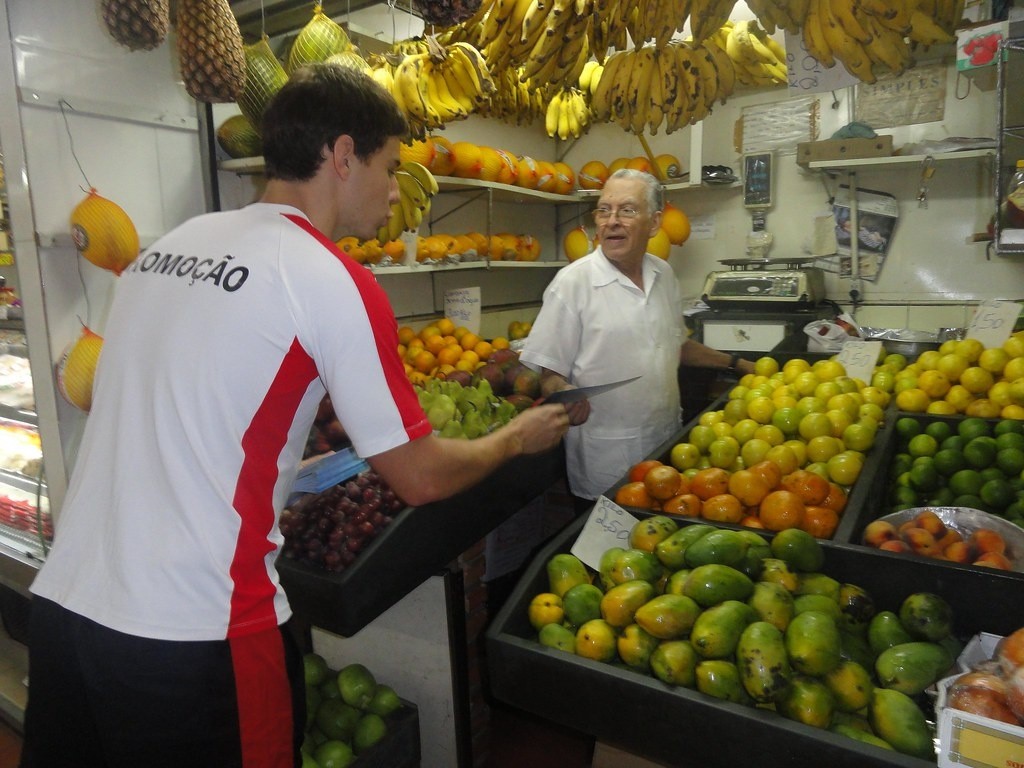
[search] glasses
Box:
[591,208,655,227]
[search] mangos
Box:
[533,512,954,755]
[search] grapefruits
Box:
[71,190,139,277]
[56,325,103,411]
[338,132,687,273]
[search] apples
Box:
[299,651,400,768]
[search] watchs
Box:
[726,353,741,377]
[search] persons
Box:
[18,63,578,768]
[517,165,761,516]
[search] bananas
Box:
[373,0,964,140]
[368,161,437,242]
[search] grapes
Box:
[276,471,404,568]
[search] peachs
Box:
[865,506,1009,575]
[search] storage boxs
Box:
[0,332,1024,768]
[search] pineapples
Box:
[101,0,247,101]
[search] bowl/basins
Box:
[865,505,1024,576]
[869,337,942,357]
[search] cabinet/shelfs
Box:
[215,1,1022,276]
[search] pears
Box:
[402,366,516,437]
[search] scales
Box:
[699,248,838,313]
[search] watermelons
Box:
[221,16,366,157]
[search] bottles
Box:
[1006,159,1024,228]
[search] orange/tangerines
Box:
[618,455,841,533]
[894,410,1023,526]
[675,333,1023,475]
[387,312,507,376]
